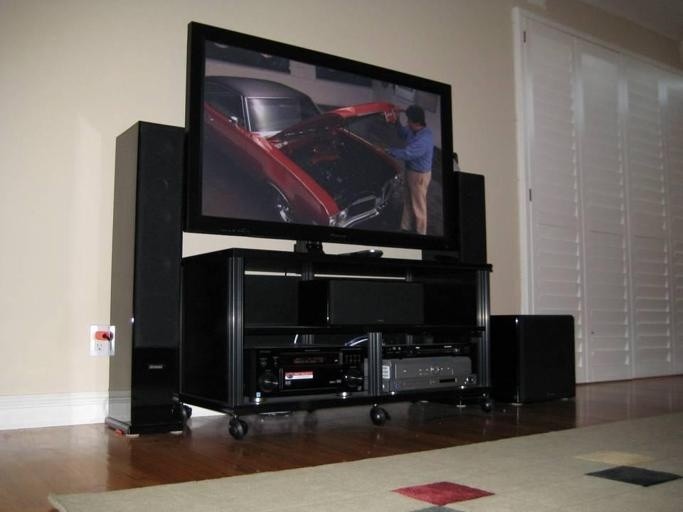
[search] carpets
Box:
[44,404,683,512]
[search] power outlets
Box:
[89,324,112,356]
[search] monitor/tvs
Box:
[183,21,455,258]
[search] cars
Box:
[203,75,408,228]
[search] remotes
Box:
[338,249,383,258]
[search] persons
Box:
[376,104,435,235]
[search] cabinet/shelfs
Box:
[180,246,495,440]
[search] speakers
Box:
[490,315,575,403]
[298,279,425,326]
[108,121,185,428]
[423,171,486,265]
[244,276,299,326]
